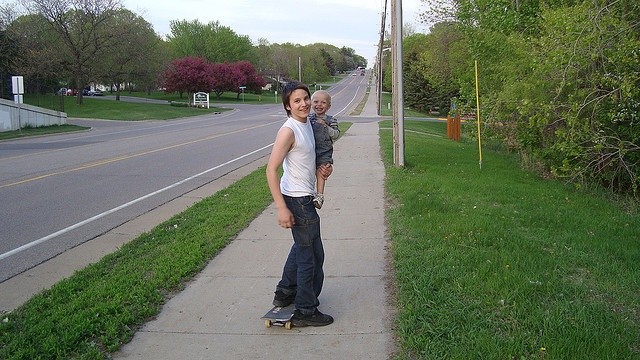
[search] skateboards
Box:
[260,301,296,330]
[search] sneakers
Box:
[291,308,334,327]
[272,291,296,307]
[313,193,324,210]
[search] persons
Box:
[308,90,340,209]
[265,81,333,328]
